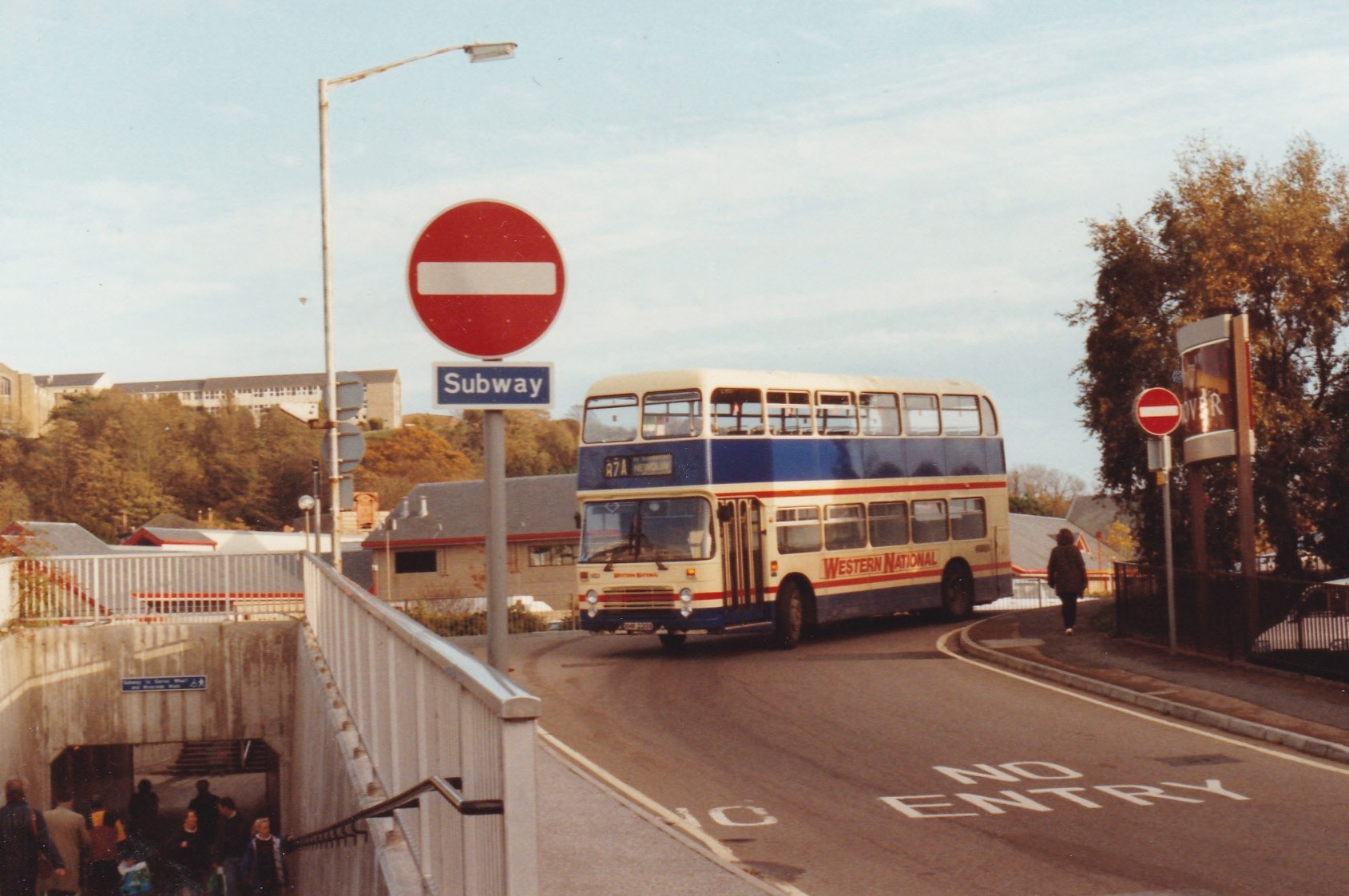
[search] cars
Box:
[1252,576,1349,679]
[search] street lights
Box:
[299,495,313,551]
[318,43,522,569]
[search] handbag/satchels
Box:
[37,852,55,879]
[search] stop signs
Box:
[1135,387,1183,437]
[405,199,566,360]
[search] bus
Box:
[573,365,1017,648]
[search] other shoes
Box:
[1064,626,1075,636]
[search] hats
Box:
[1055,528,1075,546]
[5,779,28,803]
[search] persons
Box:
[0,778,295,896]
[1048,529,1088,635]
